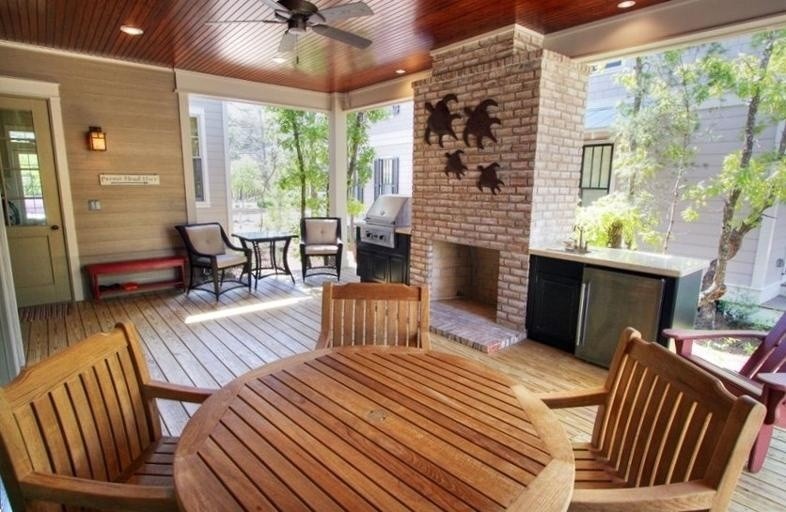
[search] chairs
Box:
[663,310,786,473]
[535,327,767,512]
[174,221,253,302]
[315,282,432,352]
[0,320,219,512]
[298,217,343,283]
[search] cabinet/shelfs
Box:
[355,223,411,284]
[524,253,702,372]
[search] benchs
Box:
[84,256,188,305]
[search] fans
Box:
[204,1,374,53]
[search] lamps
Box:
[286,14,307,35]
[89,126,106,151]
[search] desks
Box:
[230,231,299,291]
[174,345,576,512]
